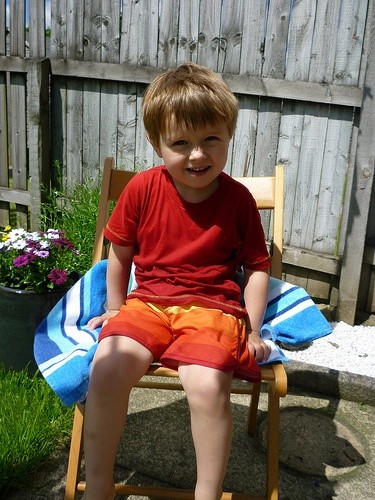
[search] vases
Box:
[0,285,51,348]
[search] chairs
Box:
[65,157,287,500]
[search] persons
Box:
[81,64,272,500]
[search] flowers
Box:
[0,225,80,292]
